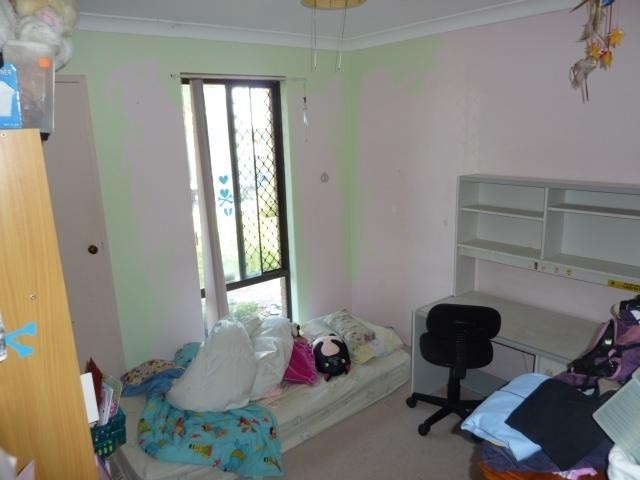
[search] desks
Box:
[478,445,610,480]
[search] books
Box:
[79,355,125,427]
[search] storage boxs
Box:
[1,40,57,139]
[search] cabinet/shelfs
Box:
[410,294,605,400]
[452,173,640,292]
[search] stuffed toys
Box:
[0,0,79,75]
[310,332,352,382]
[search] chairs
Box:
[406,303,501,444]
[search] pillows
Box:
[164,317,257,414]
[242,314,294,402]
[119,358,184,388]
[321,308,376,350]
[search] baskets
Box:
[89,406,130,460]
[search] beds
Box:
[110,308,413,480]
